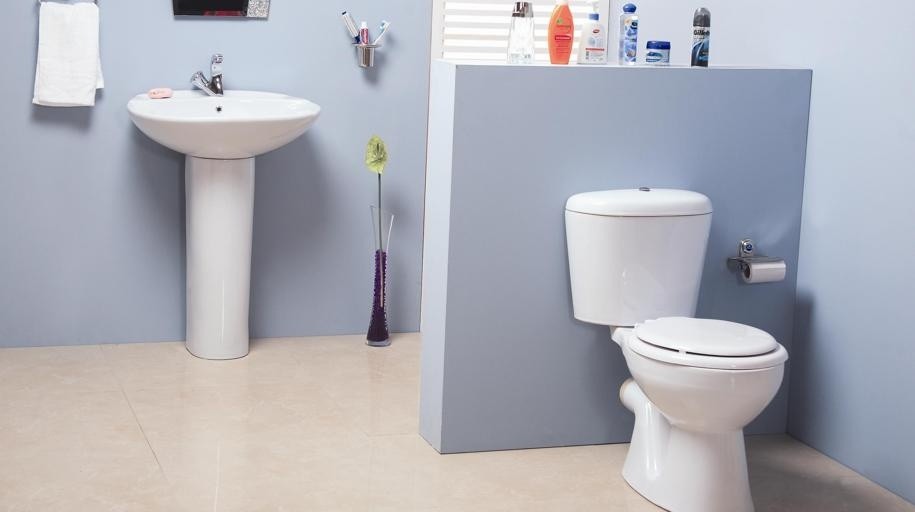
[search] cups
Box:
[357,46,374,68]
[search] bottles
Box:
[505,2,534,63]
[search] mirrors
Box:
[172,1,271,19]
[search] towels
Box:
[31,0,104,106]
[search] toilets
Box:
[561,185,787,511]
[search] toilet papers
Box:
[740,259,788,286]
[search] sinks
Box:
[127,90,322,160]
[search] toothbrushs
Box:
[372,19,391,46]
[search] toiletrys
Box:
[547,2,575,61]
[359,21,369,45]
[581,2,610,66]
[691,8,710,65]
[508,2,538,63]
[618,4,638,66]
[646,40,670,66]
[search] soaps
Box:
[148,86,173,99]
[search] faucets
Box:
[207,53,225,92]
